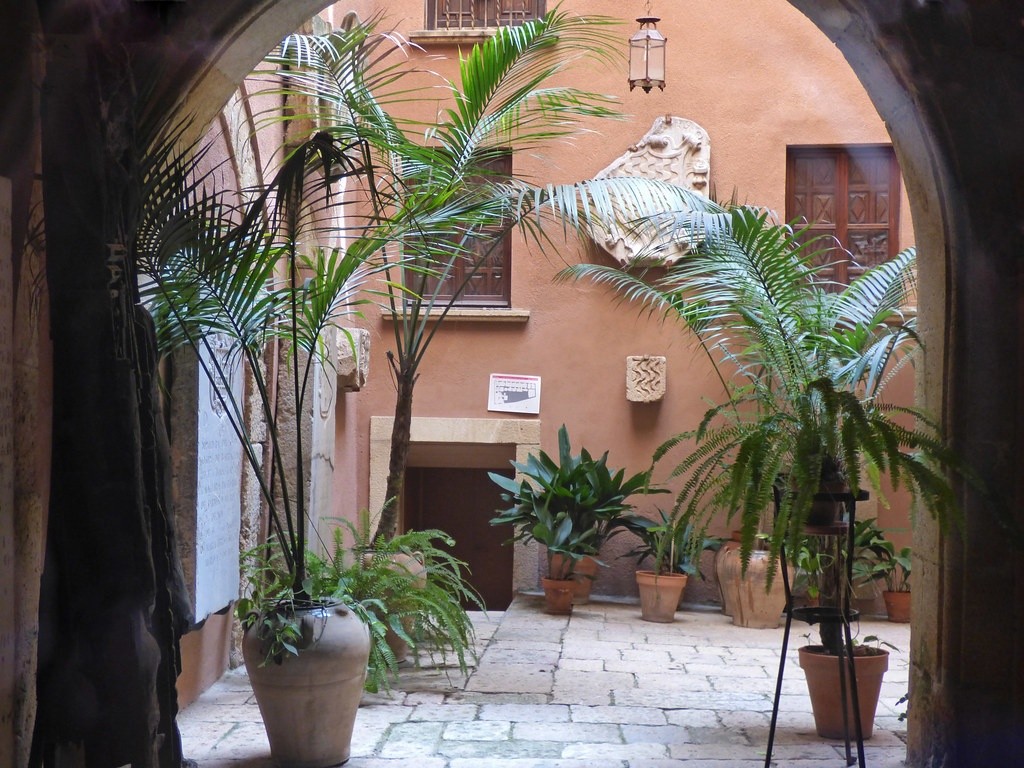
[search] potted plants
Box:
[487,425,620,614]
[857,545,912,622]
[302,0,636,664]
[135,126,475,766]
[549,439,673,602]
[612,502,723,623]
[796,632,901,739]
[799,252,848,523]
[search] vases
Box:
[716,530,747,618]
[763,483,870,768]
[718,546,796,628]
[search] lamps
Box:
[628,0,668,92]
